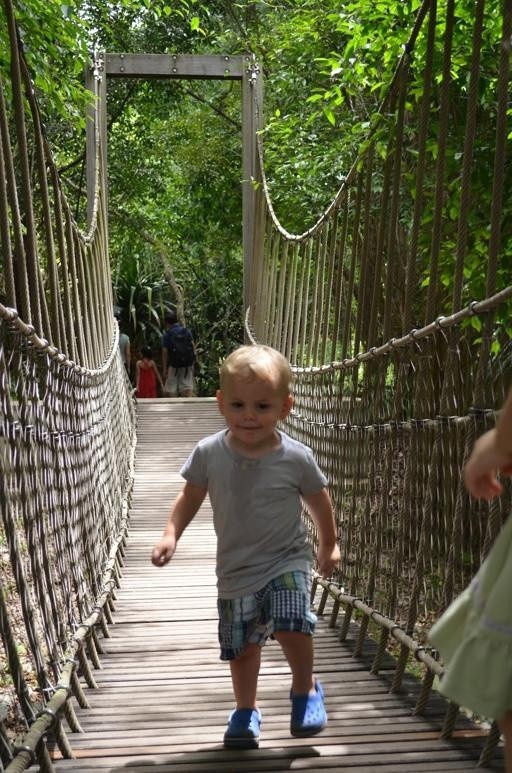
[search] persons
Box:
[428,386,512,773]
[135,347,163,398]
[114,314,131,375]
[150,343,341,750]
[162,312,195,397]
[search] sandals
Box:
[289,677,327,736]
[224,705,262,748]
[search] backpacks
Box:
[166,328,196,366]
